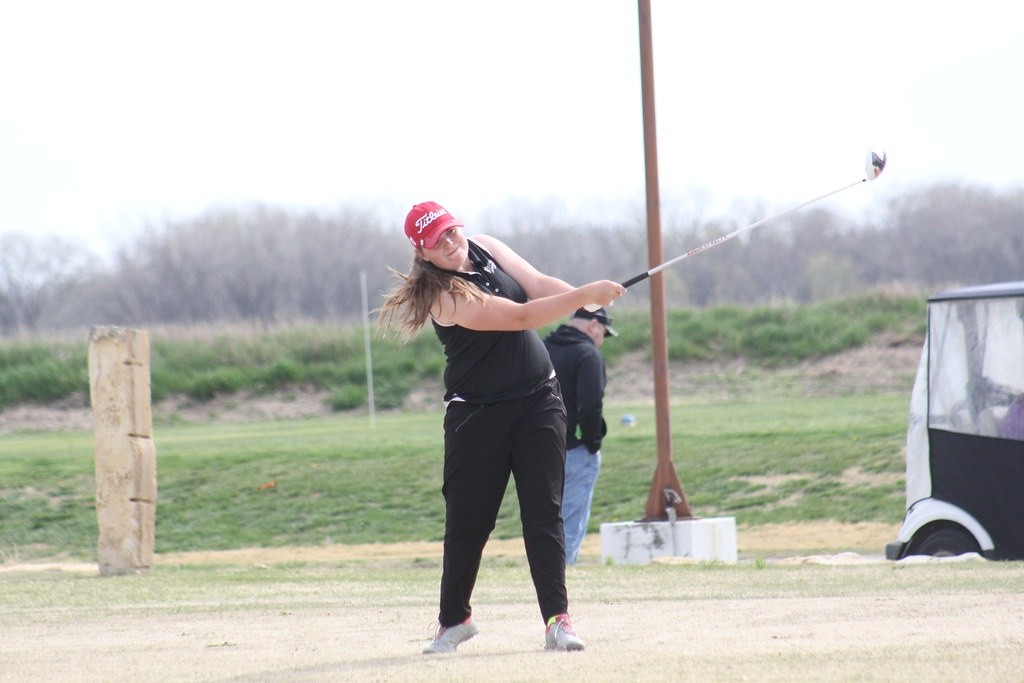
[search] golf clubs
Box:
[620,146,888,289]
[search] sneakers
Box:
[422,615,480,654]
[565,566,577,578]
[544,613,586,650]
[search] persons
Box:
[375,201,627,651]
[543,302,620,570]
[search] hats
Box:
[404,201,463,249]
[575,304,619,338]
